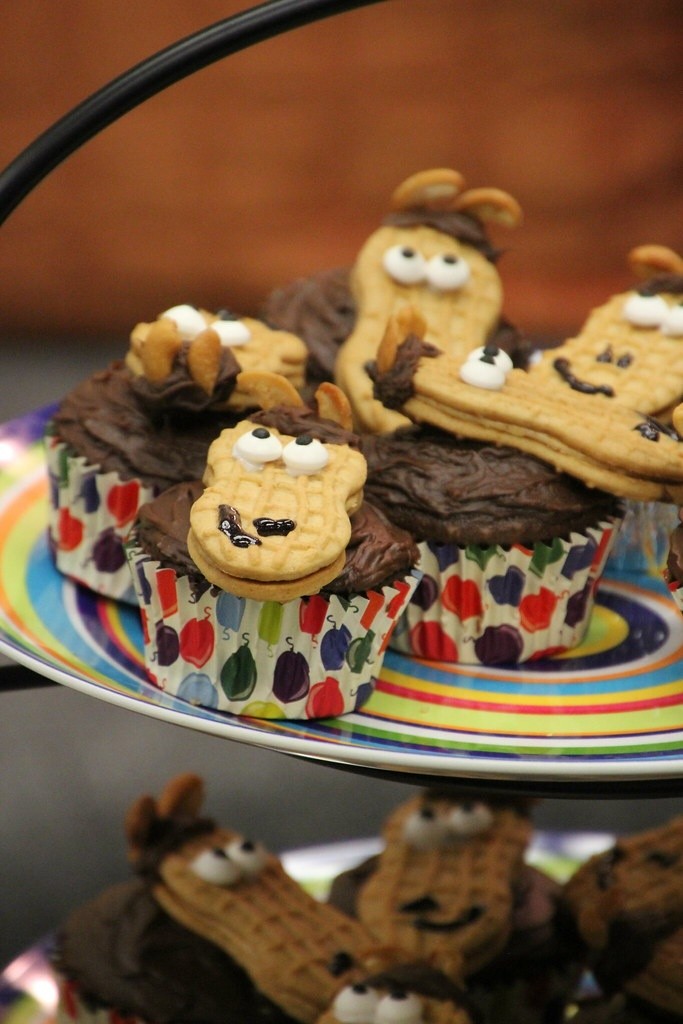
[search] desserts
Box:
[40,169,682,719]
[45,779,683,1024]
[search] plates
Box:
[0,831,683,1024]
[0,395,683,789]
